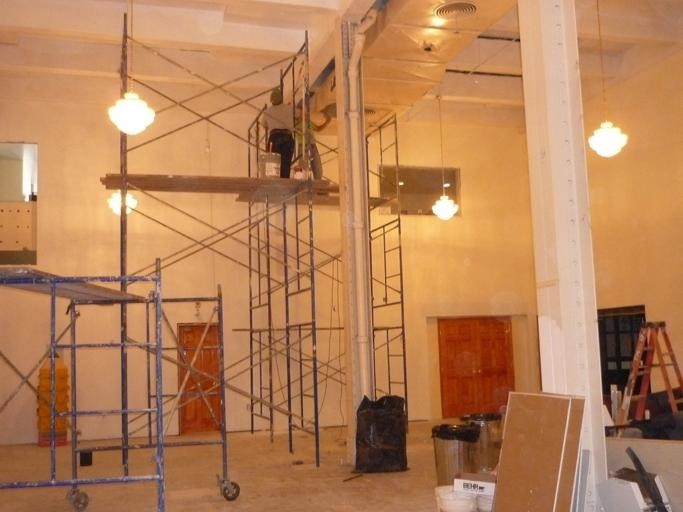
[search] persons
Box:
[295,107,331,181]
[261,89,302,179]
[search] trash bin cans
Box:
[461,414,502,471]
[432,422,481,486]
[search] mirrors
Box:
[1,142,40,202]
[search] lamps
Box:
[107,1,157,137]
[432,92,459,220]
[587,0,628,157]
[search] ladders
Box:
[621,320,683,421]
